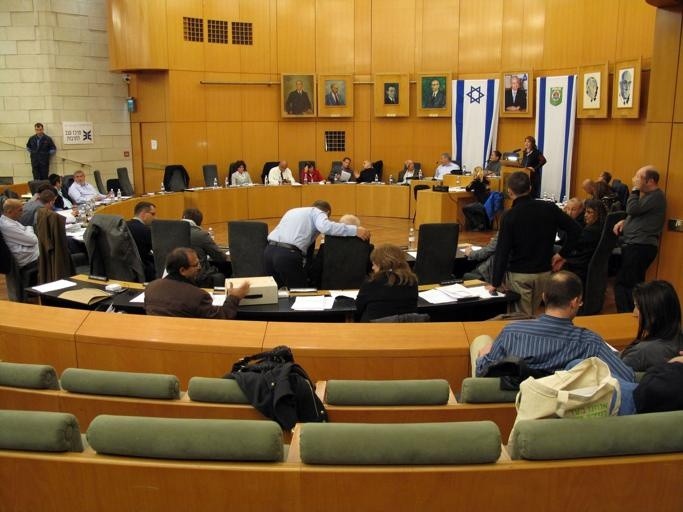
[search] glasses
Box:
[187,262,200,268]
[145,211,156,216]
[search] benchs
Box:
[2,357,679,512]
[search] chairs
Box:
[582,173,631,315]
[261,162,281,184]
[371,159,384,182]
[0,230,39,305]
[36,208,88,283]
[319,234,371,291]
[298,160,315,182]
[163,164,190,191]
[227,220,270,279]
[330,160,343,168]
[83,213,147,282]
[228,162,248,188]
[0,165,135,207]
[415,222,461,284]
[152,220,189,282]
[403,161,421,172]
[413,183,430,224]
[202,164,221,187]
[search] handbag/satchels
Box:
[506,355,622,454]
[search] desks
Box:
[416,185,482,231]
[74,311,268,383]
[246,182,301,219]
[57,189,184,248]
[442,174,502,228]
[301,182,356,216]
[1,298,91,372]
[463,311,650,373]
[357,181,410,220]
[262,320,471,393]
[185,188,246,224]
[1,273,507,324]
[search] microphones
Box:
[512,148,521,153]
[431,160,438,180]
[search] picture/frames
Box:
[611,55,642,120]
[415,72,452,118]
[373,73,409,119]
[316,73,353,118]
[574,60,610,120]
[279,72,317,119]
[497,67,534,120]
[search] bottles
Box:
[540,192,567,207]
[212,178,218,189]
[78,188,121,225]
[262,173,393,186]
[407,227,415,249]
[160,182,165,195]
[223,176,229,188]
[207,227,214,240]
[415,164,467,191]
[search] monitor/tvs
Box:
[503,153,519,161]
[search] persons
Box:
[300,161,324,184]
[610,280,682,373]
[327,83,344,105]
[328,157,380,183]
[521,136,547,199]
[618,71,632,106]
[230,160,253,185]
[125,201,233,288]
[433,153,460,180]
[397,160,423,182]
[356,244,419,323]
[505,76,527,112]
[384,84,398,103]
[1,170,111,272]
[262,200,374,292]
[463,166,491,222]
[268,160,295,184]
[26,123,56,180]
[286,80,313,114]
[490,165,667,317]
[425,80,446,108]
[145,247,250,319]
[468,270,635,385]
[584,77,601,108]
[486,151,500,171]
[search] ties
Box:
[279,172,284,180]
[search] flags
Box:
[452,78,501,175]
[534,75,578,204]
